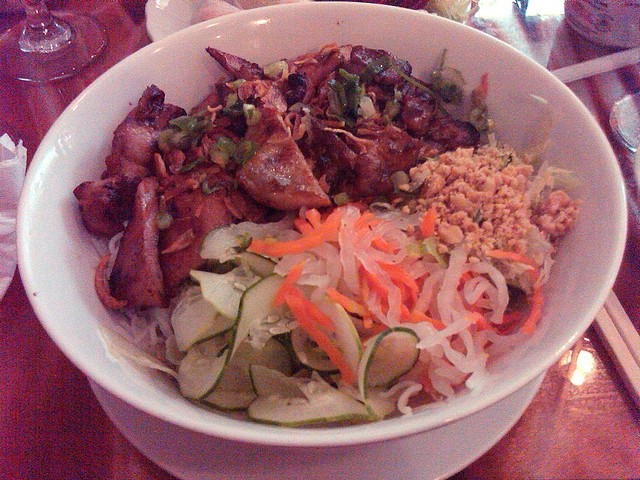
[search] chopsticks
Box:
[590,287,640,411]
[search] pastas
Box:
[90,218,176,356]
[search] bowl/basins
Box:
[13,1,629,448]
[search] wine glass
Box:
[0,0,108,84]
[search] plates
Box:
[86,370,546,480]
